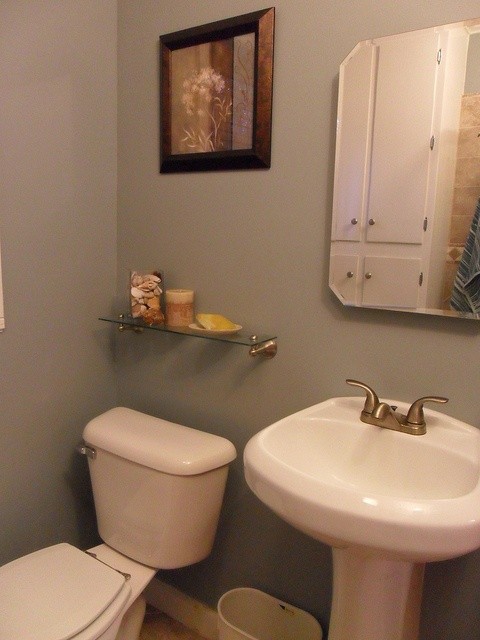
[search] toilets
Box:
[0,406,237,640]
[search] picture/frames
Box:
[158,5,276,175]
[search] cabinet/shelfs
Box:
[97,311,279,360]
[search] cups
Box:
[165,289,194,327]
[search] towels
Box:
[449,196,480,315]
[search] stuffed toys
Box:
[131,274,163,317]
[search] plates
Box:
[188,323,242,336]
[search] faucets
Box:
[346,379,449,434]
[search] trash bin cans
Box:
[218,586,323,640]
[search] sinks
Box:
[243,398,479,562]
